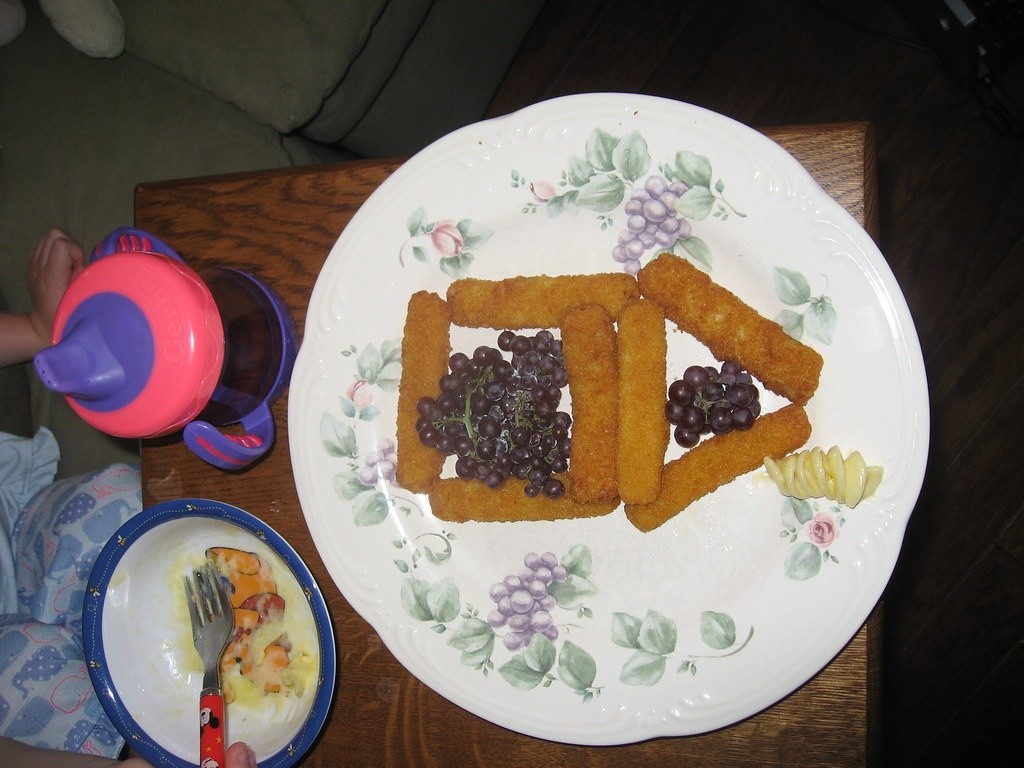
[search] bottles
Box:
[32,225,297,471]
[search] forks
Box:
[183,560,236,768]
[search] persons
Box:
[0,227,259,768]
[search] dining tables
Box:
[125,115,884,768]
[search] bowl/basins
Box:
[82,497,337,767]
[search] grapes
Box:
[416,330,573,498]
[666,359,761,448]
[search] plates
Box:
[288,91,931,746]
